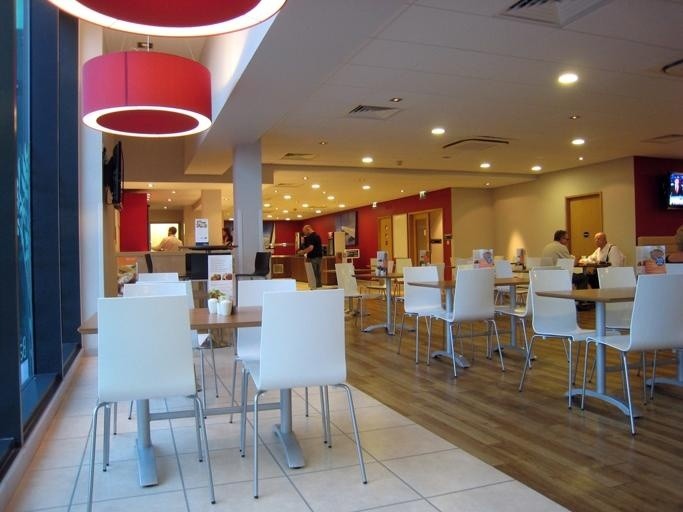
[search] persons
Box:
[671,178,683,196]
[543,229,578,307]
[668,225,683,263]
[222,227,233,246]
[637,247,666,275]
[383,253,388,268]
[584,233,623,289]
[479,252,493,268]
[298,225,323,287]
[152,227,183,251]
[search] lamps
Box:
[48,0,288,40]
[78,31,213,140]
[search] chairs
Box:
[70,249,368,509]
[516,267,680,434]
[335,249,682,378]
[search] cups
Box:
[580,255,600,265]
[219,301,231,316]
[207,299,218,313]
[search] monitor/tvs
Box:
[665,170,683,210]
[105,139,125,212]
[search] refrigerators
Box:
[327,231,345,256]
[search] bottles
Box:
[419,255,425,266]
